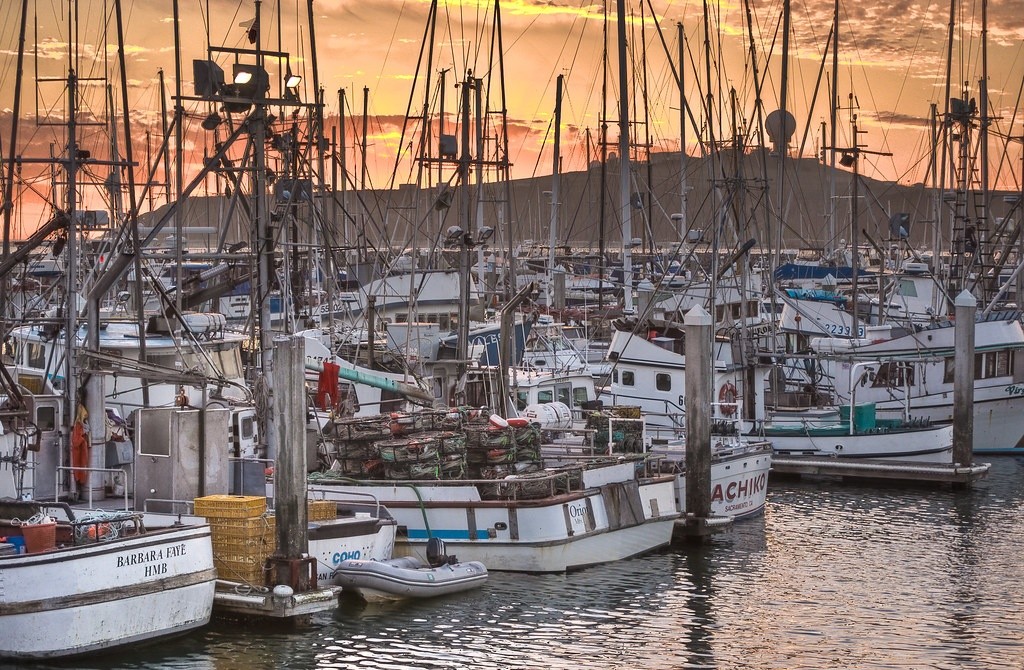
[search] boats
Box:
[0,0,1024,664]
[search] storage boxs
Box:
[194,494,336,585]
[840,401,876,432]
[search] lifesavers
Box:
[719,382,737,413]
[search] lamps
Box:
[232,68,253,85]
[446,225,463,238]
[478,226,494,244]
[284,74,302,87]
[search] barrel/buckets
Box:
[19,521,56,553]
[6,536,23,554]
